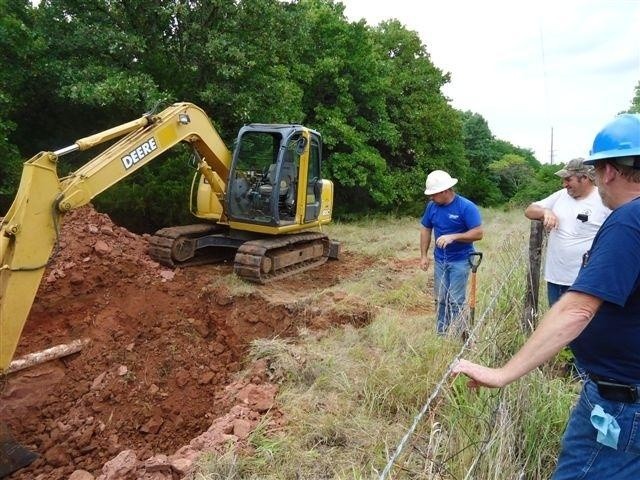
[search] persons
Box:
[447,108,639,479]
[521,156,615,387]
[418,169,485,340]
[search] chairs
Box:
[258,149,296,197]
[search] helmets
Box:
[422,168,460,197]
[580,113,640,166]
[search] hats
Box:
[552,157,597,181]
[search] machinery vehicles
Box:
[0,100,335,377]
